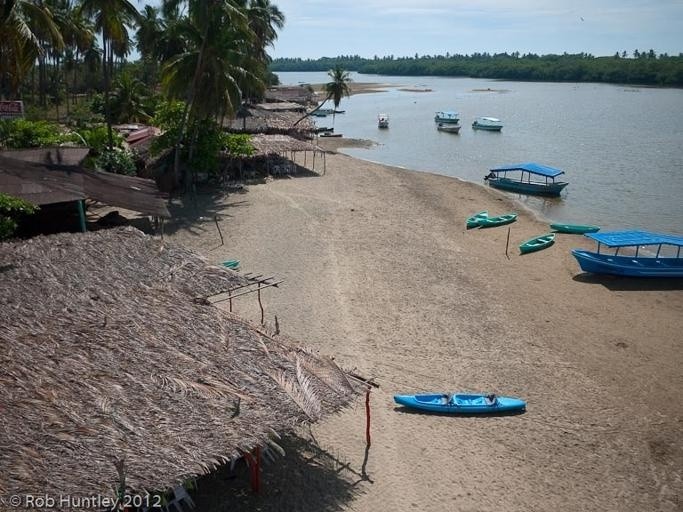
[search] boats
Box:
[378,113,390,128]
[391,391,528,416]
[568,229,683,279]
[483,162,570,198]
[465,210,600,254]
[434,110,504,134]
